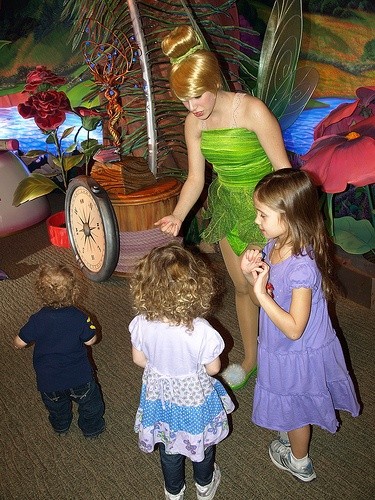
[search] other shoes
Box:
[82,423,105,437]
[53,423,69,431]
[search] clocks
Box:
[64,176,121,283]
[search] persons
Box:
[240,168,361,483]
[128,241,236,500]
[154,23,292,390]
[13,261,105,437]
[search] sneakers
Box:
[164,479,185,499]
[269,430,317,483]
[195,462,221,500]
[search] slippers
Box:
[222,361,258,389]
